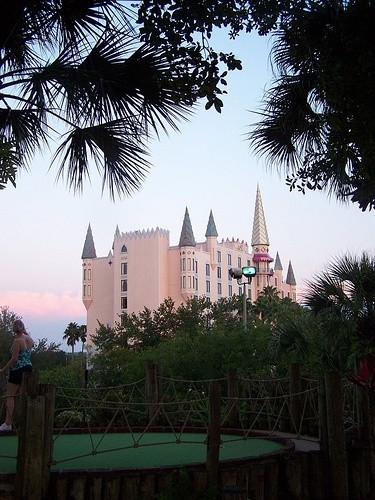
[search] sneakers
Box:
[0,423,12,430]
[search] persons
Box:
[0,320,35,431]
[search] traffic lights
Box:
[229,265,257,285]
